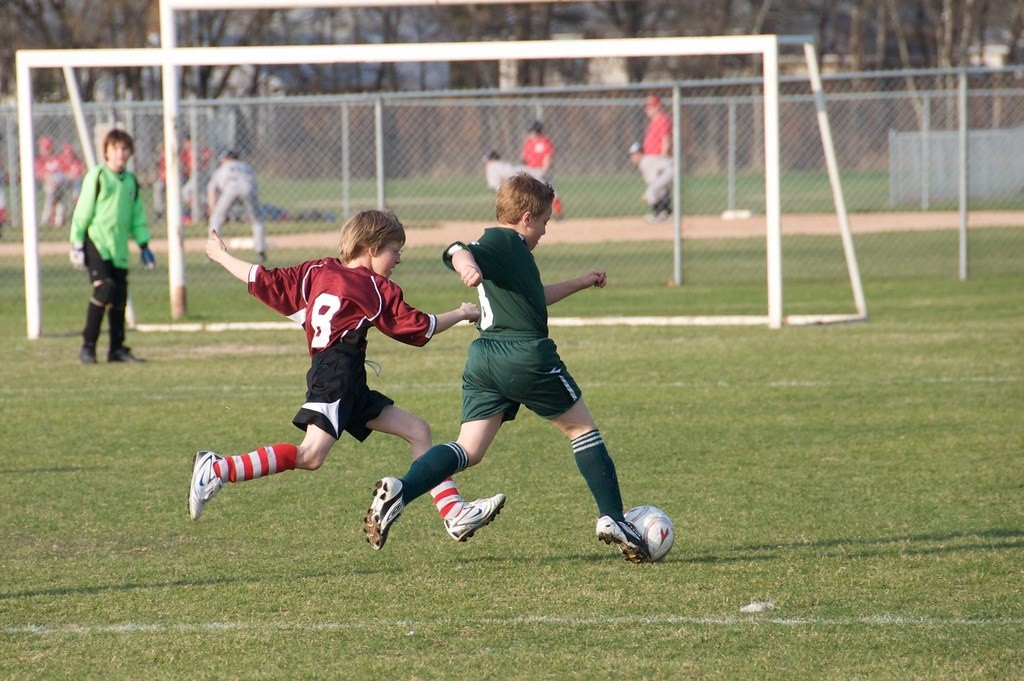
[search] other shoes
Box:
[80,343,98,365]
[107,349,146,365]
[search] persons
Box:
[482,119,565,221]
[364,172,652,565]
[0,132,267,264]
[70,129,157,365]
[627,97,672,226]
[187,211,505,541]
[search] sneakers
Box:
[595,513,650,564]
[187,451,223,523]
[364,477,405,551]
[444,494,507,542]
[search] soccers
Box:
[623,505,676,564]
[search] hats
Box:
[483,150,501,160]
[218,148,240,159]
[525,121,543,133]
[643,95,661,107]
[626,143,644,155]
